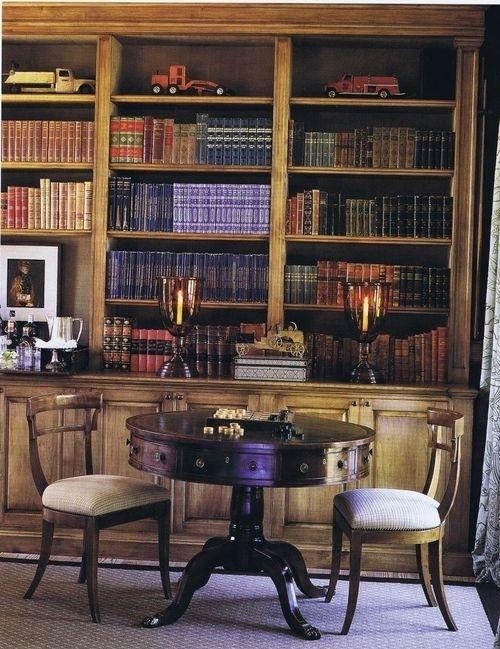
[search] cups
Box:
[12,345,41,374]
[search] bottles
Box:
[22,315,37,338]
[5,309,18,349]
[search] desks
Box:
[119,407,377,639]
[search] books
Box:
[105,318,450,384]
[286,189,454,238]
[107,178,271,234]
[2,120,95,162]
[104,250,270,303]
[110,116,273,165]
[285,261,451,308]
[1,178,92,229]
[288,120,456,171]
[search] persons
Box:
[10,259,37,308]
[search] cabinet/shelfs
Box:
[1,2,492,583]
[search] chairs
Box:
[21,386,179,624]
[323,408,466,633]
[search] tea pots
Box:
[46,315,83,342]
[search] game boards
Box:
[207,409,294,430]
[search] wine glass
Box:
[154,276,203,379]
[340,282,391,383]
